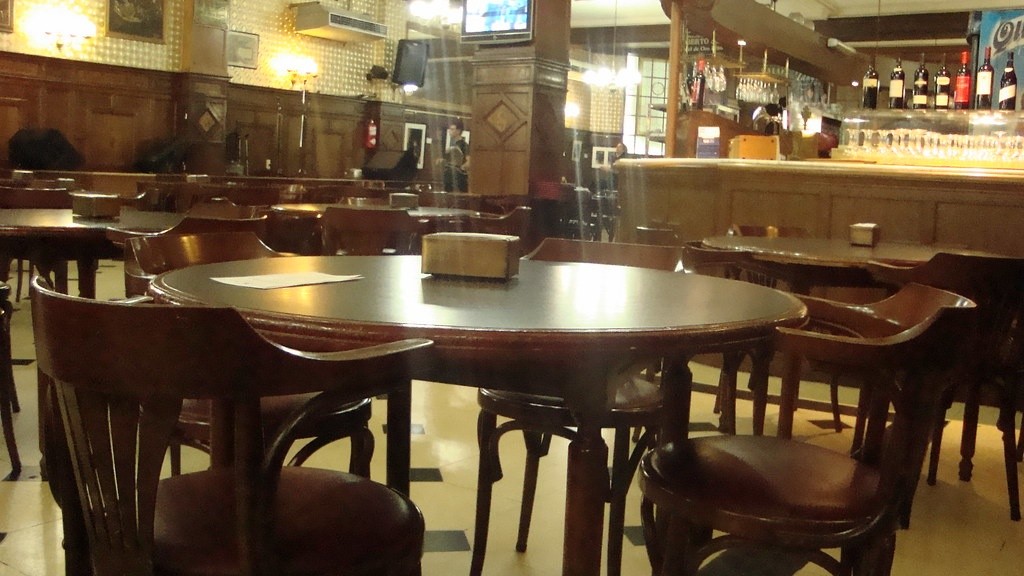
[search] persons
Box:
[434,121,470,195]
[605,143,629,192]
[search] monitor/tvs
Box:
[460,0,535,44]
[392,39,430,87]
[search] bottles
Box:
[953,52,972,110]
[913,53,929,109]
[681,60,705,111]
[933,54,951,109]
[889,54,906,109]
[862,51,879,109]
[974,47,995,110]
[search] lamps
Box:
[366,64,388,81]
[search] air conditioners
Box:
[295,3,389,43]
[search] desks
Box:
[0,208,186,299]
[702,235,1012,466]
[150,258,804,575]
[270,203,497,255]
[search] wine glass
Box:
[705,65,727,92]
[735,77,780,104]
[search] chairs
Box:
[0,184,1024,576]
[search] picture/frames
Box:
[104,0,168,45]
[226,30,260,70]
[403,123,427,169]
[0,0,14,32]
